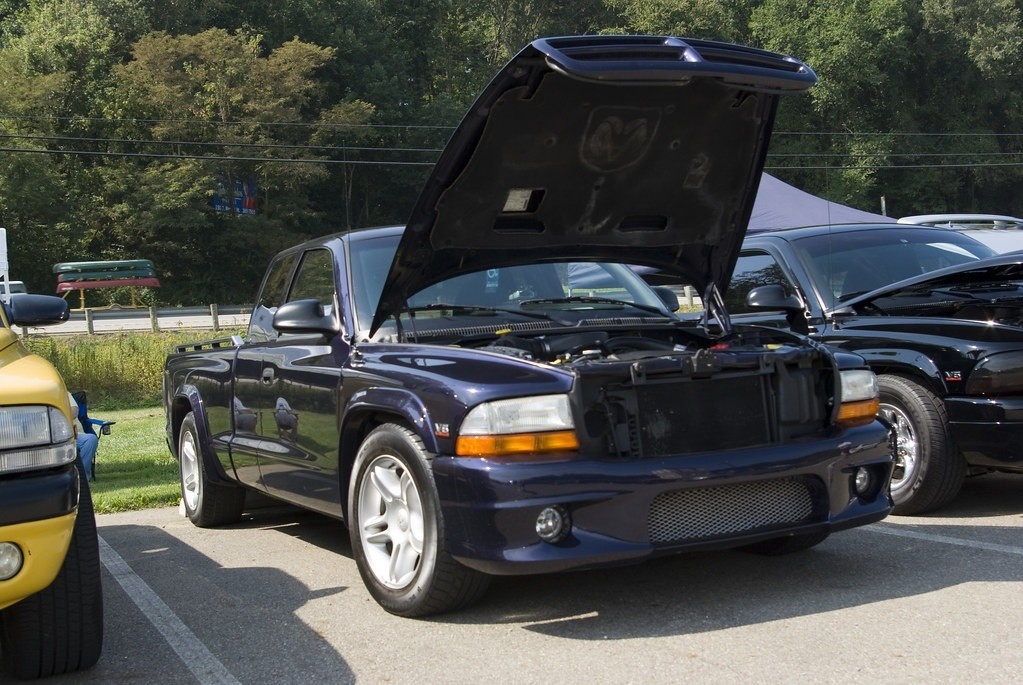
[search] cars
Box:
[841,213,1023,303]
[162,35,898,617]
[1,296,104,678]
[0,281,27,304]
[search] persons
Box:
[241,175,256,209]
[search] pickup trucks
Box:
[680,222,1023,514]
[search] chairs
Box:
[71,391,116,483]
[436,270,496,306]
[497,262,565,301]
[841,272,875,296]
